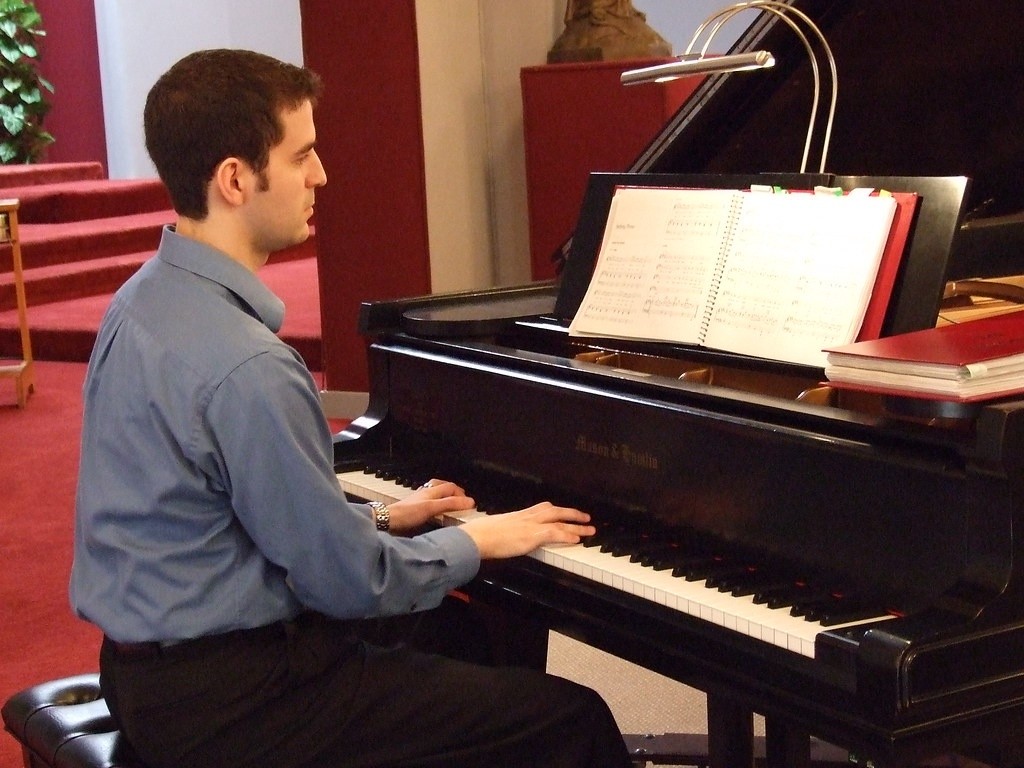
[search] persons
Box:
[70,50,634,768]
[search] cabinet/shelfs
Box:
[520,53,723,282]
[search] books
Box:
[568,181,1024,403]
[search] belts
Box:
[103,623,275,667]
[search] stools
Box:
[0,673,144,768]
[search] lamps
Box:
[620,1,838,173]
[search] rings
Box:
[424,483,433,488]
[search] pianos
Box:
[332,0,1023,768]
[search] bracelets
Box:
[367,501,389,532]
